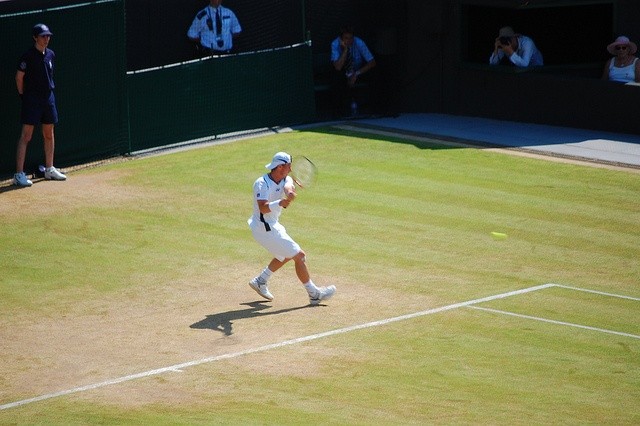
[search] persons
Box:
[330,27,376,115]
[12,24,67,186]
[489,27,544,69]
[247,152,336,305]
[601,35,640,84]
[186,0,242,54]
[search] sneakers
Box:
[309,284,336,304]
[13,170,33,185]
[45,166,66,179]
[249,278,274,299]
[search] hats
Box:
[32,23,54,35]
[607,35,637,55]
[265,151,292,169]
[497,26,519,38]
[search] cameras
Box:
[500,37,512,45]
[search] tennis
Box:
[493,232,506,240]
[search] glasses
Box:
[615,46,626,49]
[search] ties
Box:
[216,8,221,35]
